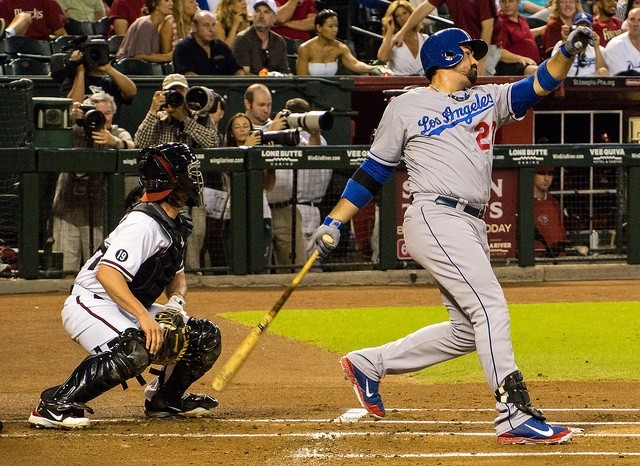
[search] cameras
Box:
[76,104,105,132]
[250,128,301,146]
[159,89,184,110]
[277,110,334,132]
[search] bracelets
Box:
[534,60,564,92]
[167,292,187,300]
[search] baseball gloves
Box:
[149,310,190,365]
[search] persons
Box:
[593,0,626,47]
[172,10,248,75]
[192,91,226,275]
[605,8,640,76]
[232,0,291,75]
[271,0,317,41]
[57,0,106,21]
[543,0,583,58]
[109,0,144,35]
[491,0,547,76]
[52,93,135,278]
[388,0,494,76]
[221,113,276,274]
[214,0,250,48]
[551,12,610,76]
[306,25,597,443]
[52,35,137,106]
[115,0,175,62]
[134,73,219,276]
[377,1,430,77]
[533,164,567,256]
[296,9,382,76]
[0,0,69,39]
[285,98,333,273]
[621,0,640,30]
[159,0,199,53]
[28,141,223,428]
[519,0,553,26]
[244,83,321,273]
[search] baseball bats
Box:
[211,234,334,392]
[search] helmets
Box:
[137,141,205,209]
[420,27,488,79]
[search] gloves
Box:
[306,215,344,263]
[558,26,595,60]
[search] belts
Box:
[267,197,294,212]
[411,192,487,219]
[69,284,104,300]
[296,199,320,208]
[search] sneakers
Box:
[29,400,91,430]
[144,392,218,418]
[497,419,574,445]
[340,353,386,419]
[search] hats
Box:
[162,73,189,91]
[572,11,593,26]
[533,163,558,174]
[254,0,278,14]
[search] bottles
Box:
[589,229,601,258]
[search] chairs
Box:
[336,36,359,75]
[523,16,547,43]
[0,34,52,74]
[108,34,122,59]
[53,36,74,71]
[94,13,114,37]
[112,58,153,78]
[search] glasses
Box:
[263,49,271,67]
[318,8,333,16]
